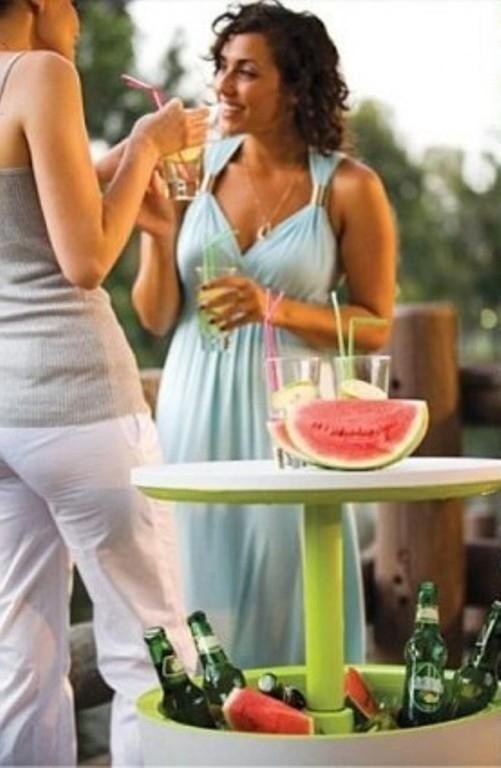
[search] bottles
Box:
[256,672,306,709]
[140,623,215,728]
[450,596,501,721]
[397,578,450,730]
[185,609,249,729]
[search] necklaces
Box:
[239,158,306,241]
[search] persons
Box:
[0,0,210,767]
[130,0,397,668]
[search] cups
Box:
[330,352,391,402]
[263,353,321,468]
[191,264,237,354]
[157,105,205,202]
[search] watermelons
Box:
[344,664,377,723]
[265,398,431,469]
[222,686,315,736]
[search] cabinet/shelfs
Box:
[108,453,501,767]
